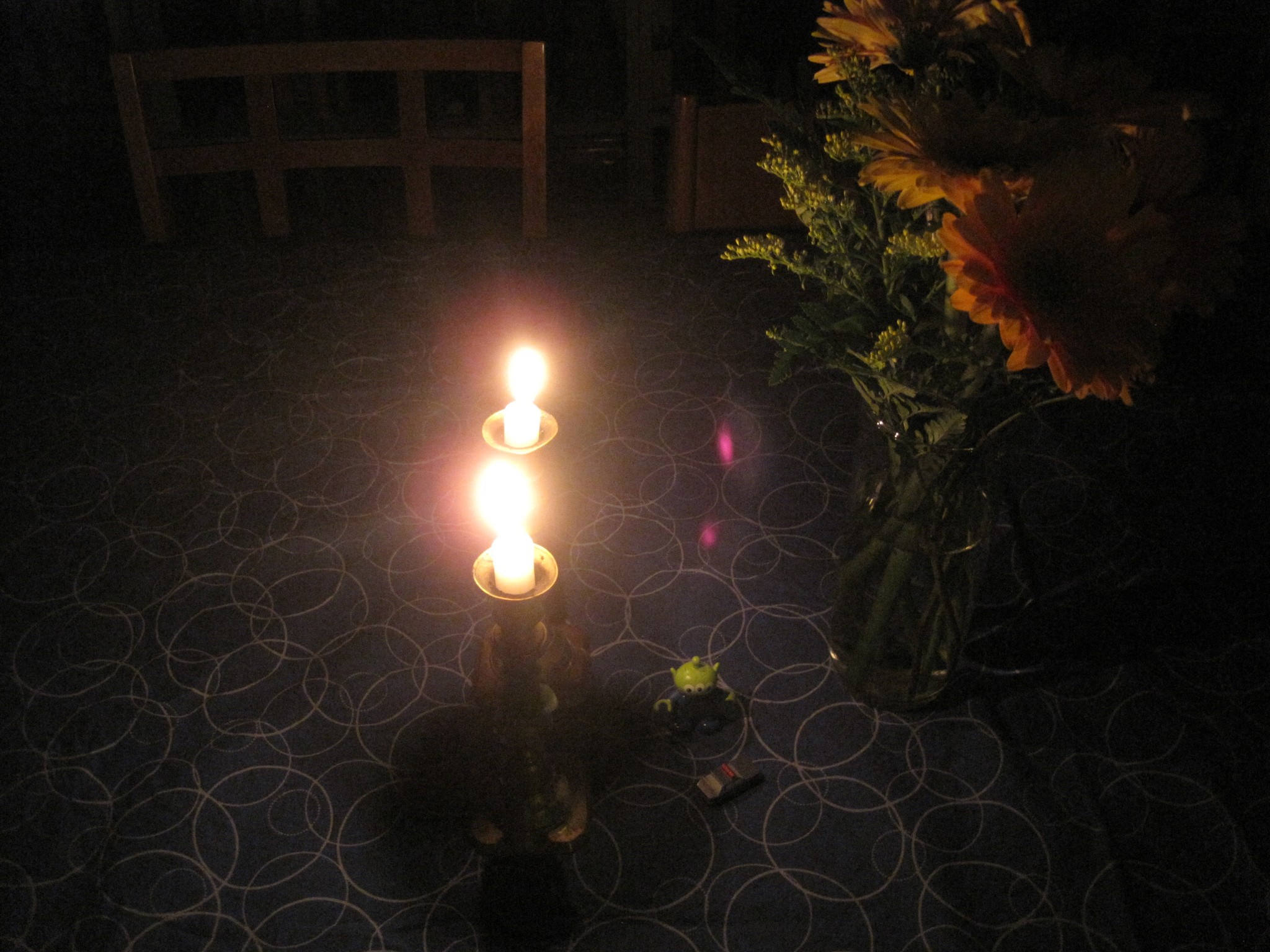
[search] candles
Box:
[470,456,544,598]
[504,343,551,448]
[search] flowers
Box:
[714,0,1215,715]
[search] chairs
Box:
[107,35,550,234]
